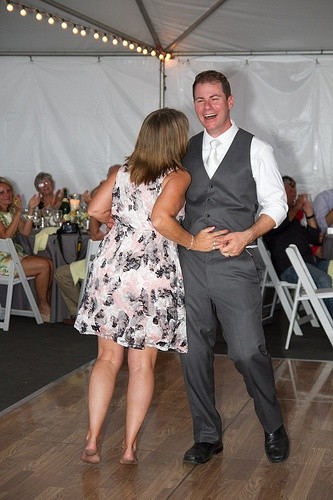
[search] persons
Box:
[262,175,333,314]
[0,172,110,326]
[82,108,229,466]
[106,69,291,465]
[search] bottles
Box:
[59,188,70,215]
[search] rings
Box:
[213,241,216,246]
[213,246,216,249]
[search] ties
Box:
[206,140,221,179]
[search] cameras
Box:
[61,221,78,233]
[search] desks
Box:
[0,224,91,323]
[315,231,333,286]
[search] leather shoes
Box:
[264,424,290,463]
[183,442,223,464]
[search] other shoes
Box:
[64,317,76,324]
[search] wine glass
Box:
[22,205,90,231]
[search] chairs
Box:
[0,235,333,350]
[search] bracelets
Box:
[306,214,314,220]
[186,234,194,252]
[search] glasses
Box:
[38,181,50,188]
